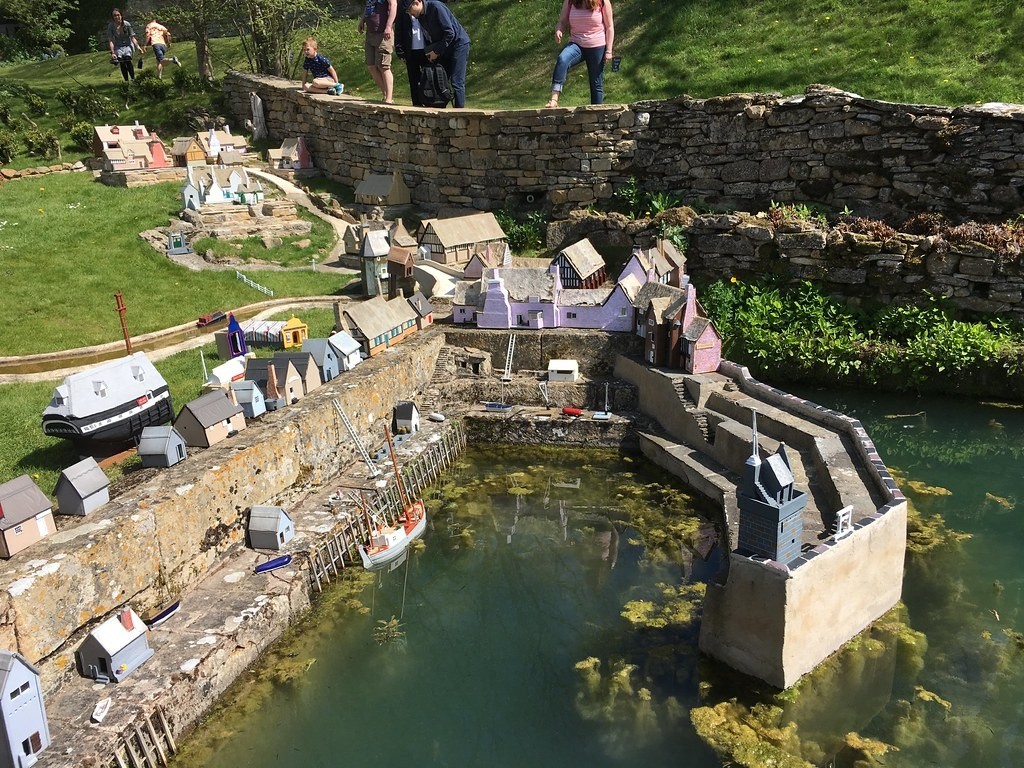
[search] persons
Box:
[107,8,143,82]
[144,15,183,80]
[302,37,344,96]
[358,0,472,108]
[545,0,614,109]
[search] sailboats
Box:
[353,422,428,570]
[484,377,515,413]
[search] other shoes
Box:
[156,75,161,79]
[382,99,394,104]
[173,57,181,67]
[544,99,558,108]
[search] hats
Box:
[399,0,414,14]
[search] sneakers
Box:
[335,83,344,95]
[327,88,337,95]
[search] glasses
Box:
[113,14,120,17]
[405,3,413,13]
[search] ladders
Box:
[347,490,389,530]
[333,399,378,476]
[504,334,516,379]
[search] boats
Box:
[252,555,292,574]
[140,596,181,632]
[428,413,445,422]
[39,288,176,462]
[561,407,582,416]
[92,697,111,722]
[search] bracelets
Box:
[605,50,612,54]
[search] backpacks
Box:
[414,56,455,108]
